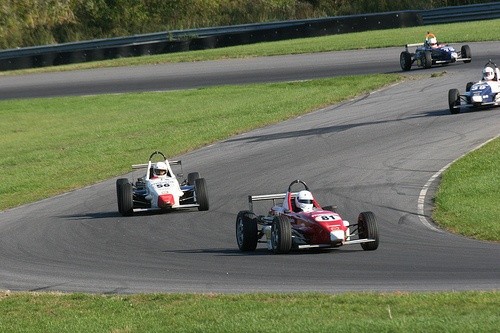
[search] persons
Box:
[481,66,495,81]
[425,33,437,48]
[151,162,168,179]
[294,190,314,213]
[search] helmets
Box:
[482,67,495,81]
[153,162,168,178]
[295,190,314,211]
[429,38,437,48]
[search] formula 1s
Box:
[448,58,500,114]
[236,180,380,255]
[116,151,210,217]
[399,32,472,72]
